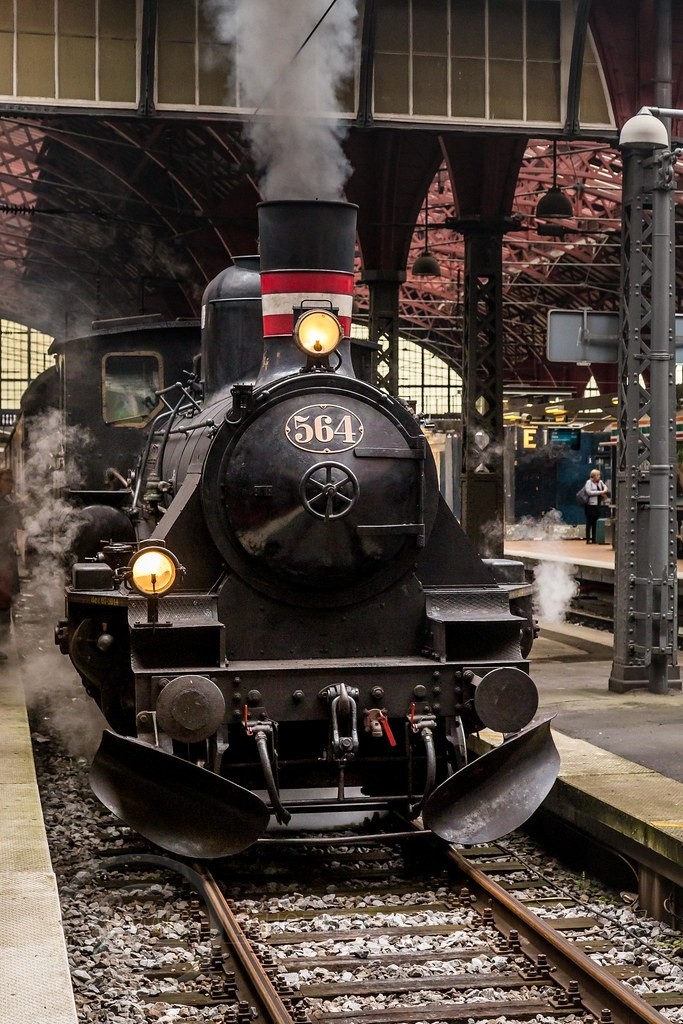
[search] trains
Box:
[8,198,562,861]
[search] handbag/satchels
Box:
[577,488,589,504]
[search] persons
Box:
[0,470,32,659]
[583,468,608,544]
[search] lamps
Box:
[618,105,683,151]
[536,139,573,218]
[412,194,441,276]
